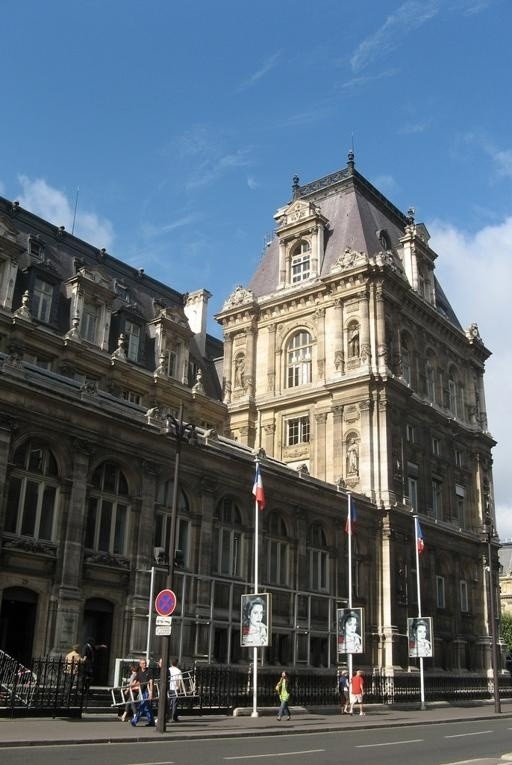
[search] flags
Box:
[417,518,428,557]
[342,498,359,537]
[252,457,271,516]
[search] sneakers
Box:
[341,711,365,715]
[117,717,155,727]
[277,716,291,721]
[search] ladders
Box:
[107,670,199,707]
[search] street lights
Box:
[481,518,502,712]
[155,398,198,734]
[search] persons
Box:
[59,642,89,707]
[241,598,269,647]
[338,669,349,716]
[274,670,293,721]
[348,670,366,716]
[336,613,363,653]
[73,636,107,695]
[116,655,182,729]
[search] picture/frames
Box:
[240,593,272,647]
[336,607,365,654]
